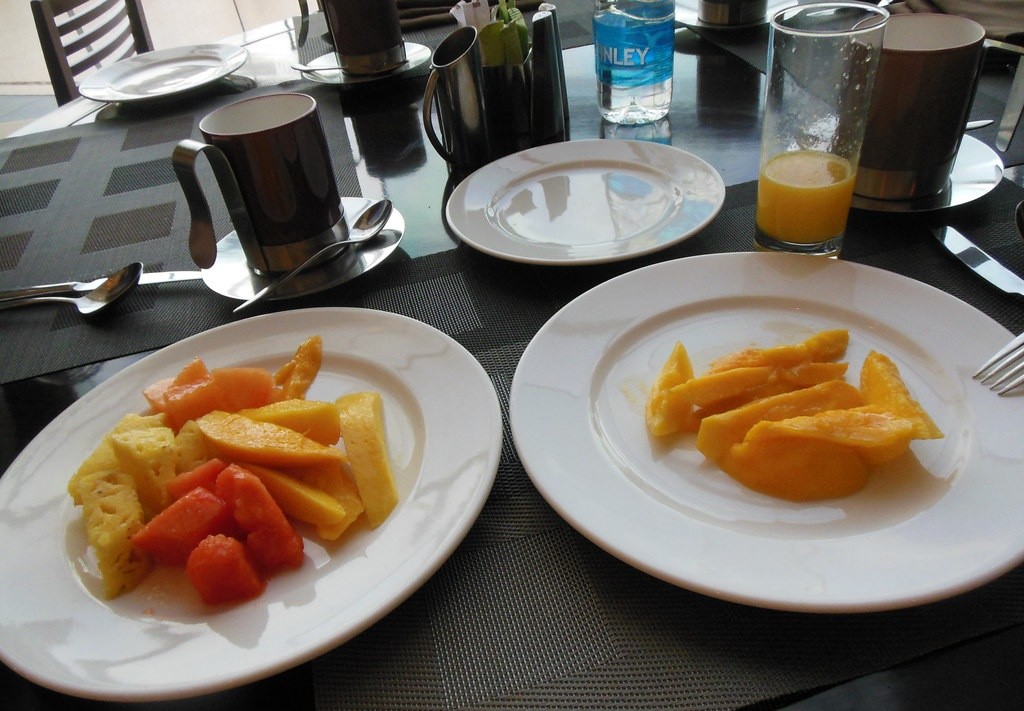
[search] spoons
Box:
[232,199,392,315]
[0,262,144,314]
[292,60,407,75]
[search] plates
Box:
[0,306,504,701]
[303,42,432,85]
[850,134,1003,213]
[445,139,726,264]
[77,43,248,102]
[200,197,405,300]
[509,252,1024,614]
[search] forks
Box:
[972,331,1024,395]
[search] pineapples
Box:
[64,412,219,599]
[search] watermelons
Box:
[130,456,305,607]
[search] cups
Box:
[853,13,986,200]
[170,92,349,279]
[752,0,890,259]
[319,0,407,77]
[697,0,767,28]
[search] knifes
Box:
[0,271,213,299]
[965,120,995,129]
[932,224,1024,297]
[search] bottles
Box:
[591,0,677,125]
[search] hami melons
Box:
[143,356,278,432]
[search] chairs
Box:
[29,0,154,109]
